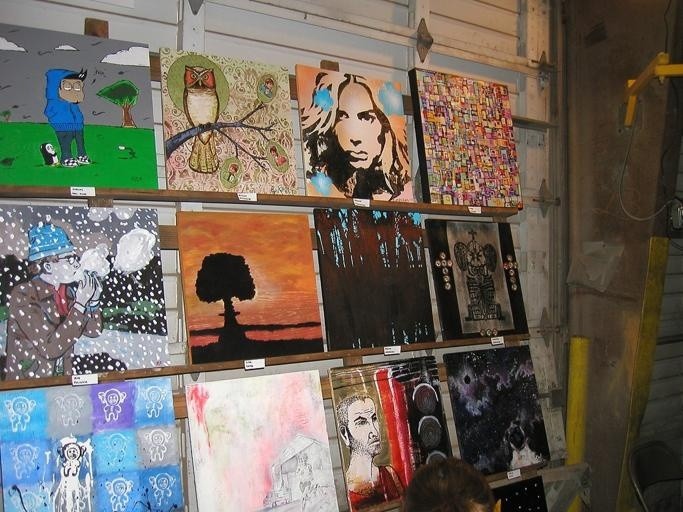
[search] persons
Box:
[44,68,92,166]
[264,78,274,98]
[6,224,103,379]
[407,459,501,512]
[270,146,287,167]
[300,73,411,200]
[227,163,239,183]
[337,393,405,512]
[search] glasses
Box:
[58,255,80,265]
[63,81,81,91]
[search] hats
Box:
[28,223,74,261]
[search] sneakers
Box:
[62,156,90,168]
